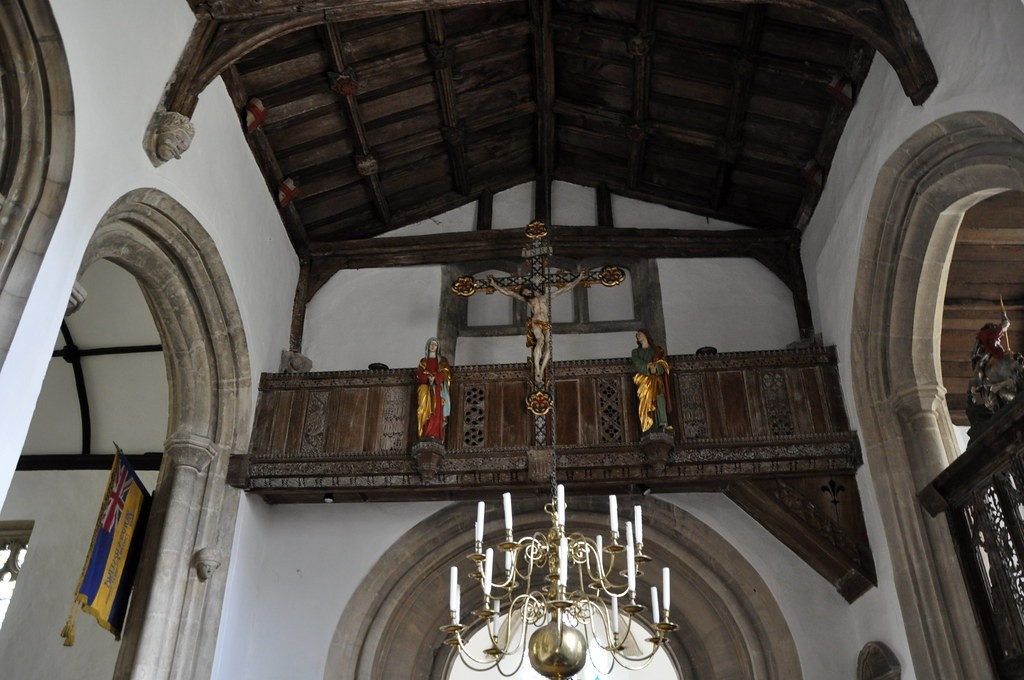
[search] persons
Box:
[485,270,588,384]
[632,329,679,434]
[970,311,1011,370]
[417,338,451,444]
[157,112,196,160]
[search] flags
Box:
[75,454,151,639]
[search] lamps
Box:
[439,107,681,680]
